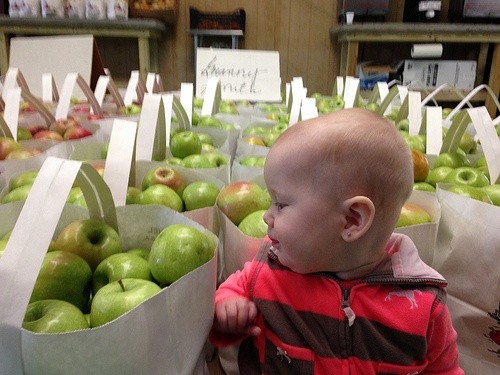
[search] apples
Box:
[0,90,500,239]
[0,219,217,333]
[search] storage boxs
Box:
[355,61,394,90]
[388,60,476,91]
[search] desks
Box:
[331,24,500,119]
[0,17,167,88]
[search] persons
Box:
[207,108,465,375]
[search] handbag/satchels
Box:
[0,153,220,375]
[0,66,500,375]
